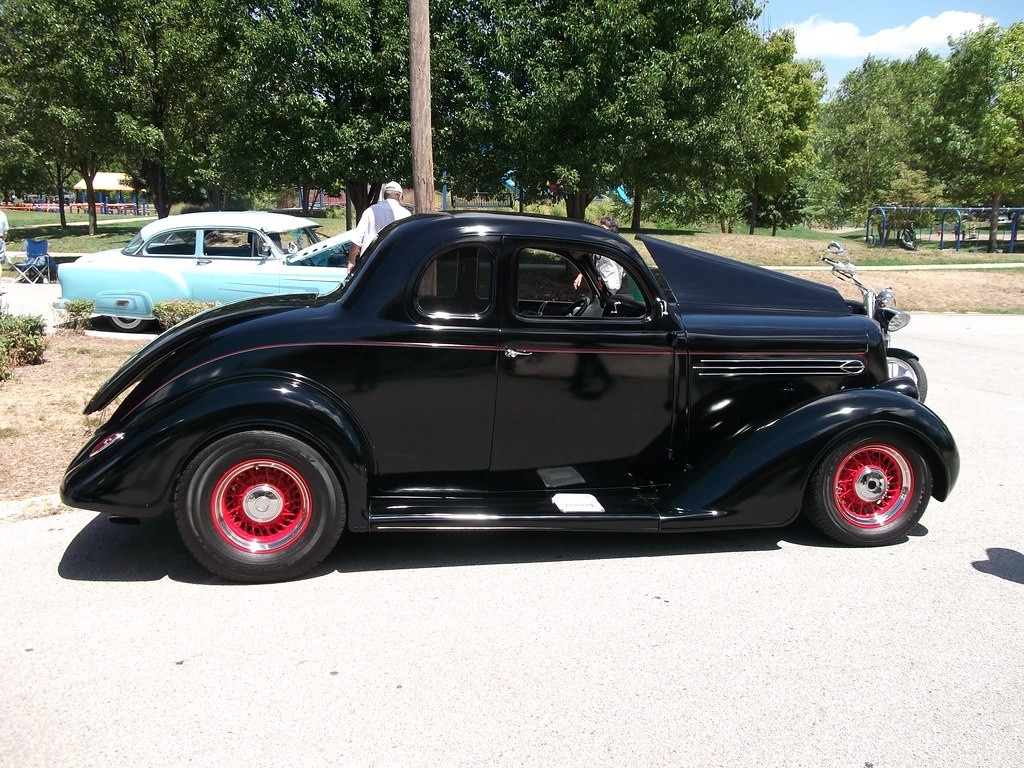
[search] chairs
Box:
[11,237,50,285]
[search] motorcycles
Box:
[820,242,927,405]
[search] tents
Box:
[74,172,146,191]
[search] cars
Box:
[53,211,358,333]
[437,246,675,305]
[60,208,960,585]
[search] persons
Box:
[954,221,959,240]
[348,181,412,277]
[573,217,626,294]
[0,211,9,295]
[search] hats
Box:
[382,181,403,193]
